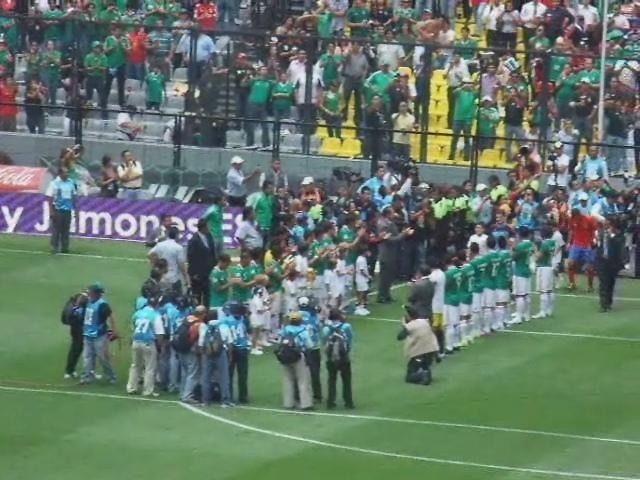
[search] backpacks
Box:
[172,318,201,353]
[61,296,82,324]
[325,327,348,371]
[203,325,223,357]
[278,328,306,364]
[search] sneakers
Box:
[523,312,530,321]
[251,348,263,355]
[201,402,210,407]
[221,402,235,408]
[503,312,522,324]
[546,309,552,316]
[423,370,431,385]
[72,372,78,378]
[64,374,70,378]
[355,306,370,316]
[531,310,546,319]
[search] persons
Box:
[1,0,272,148]
[397,160,640,385]
[79,282,118,385]
[57,145,82,176]
[45,166,78,254]
[271,1,639,160]
[117,148,398,410]
[62,289,88,380]
[100,155,119,198]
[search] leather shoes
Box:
[144,393,159,397]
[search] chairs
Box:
[12,53,190,145]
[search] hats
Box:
[299,297,309,306]
[195,305,207,312]
[230,156,245,164]
[476,183,489,191]
[91,40,103,48]
[577,192,588,201]
[289,314,301,321]
[301,177,314,185]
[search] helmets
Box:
[87,283,104,290]
